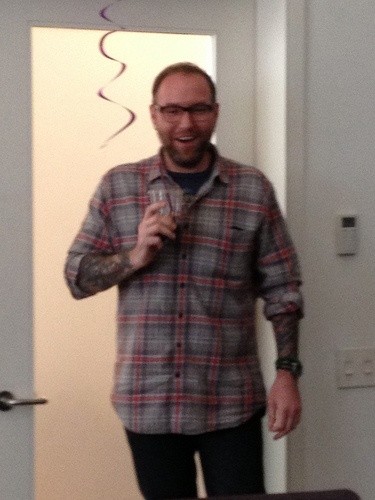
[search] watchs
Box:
[274,358,303,381]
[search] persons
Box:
[62,66,304,498]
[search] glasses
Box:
[153,101,218,123]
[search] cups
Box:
[149,179,182,247]
